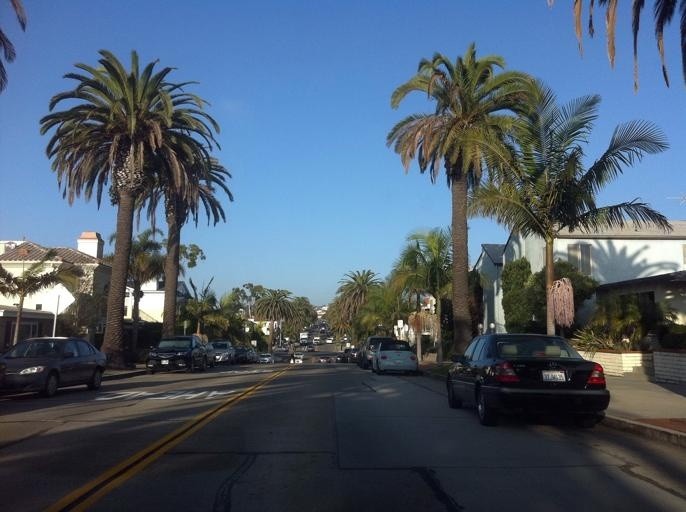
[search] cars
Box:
[0,336,106,400]
[258,321,360,364]
[369,341,417,375]
[143,335,257,375]
[443,333,610,428]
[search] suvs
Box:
[356,336,393,369]
